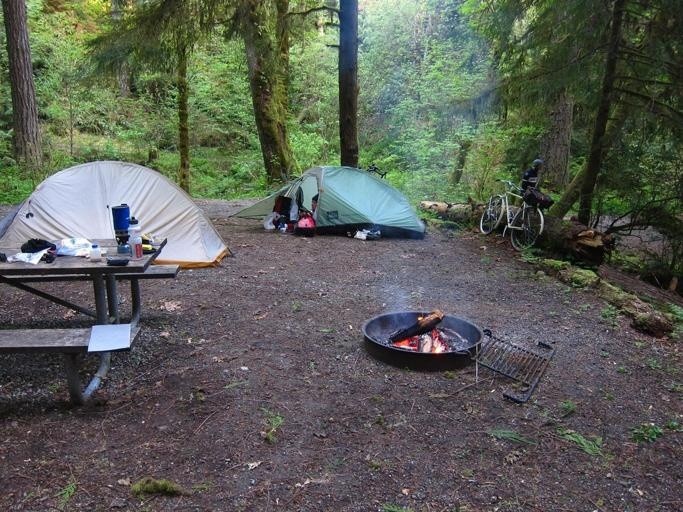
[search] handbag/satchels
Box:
[523,188,554,209]
[272,196,291,228]
[293,213,315,237]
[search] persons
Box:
[311,194,318,212]
[521,159,544,192]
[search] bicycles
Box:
[355,160,388,181]
[475,178,552,252]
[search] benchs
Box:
[0,264,180,329]
[0,324,132,406]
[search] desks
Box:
[0,236,167,406]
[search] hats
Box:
[19,239,55,253]
[533,160,542,167]
[311,193,318,203]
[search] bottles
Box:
[90,244,102,262]
[111,204,131,254]
[126,216,142,259]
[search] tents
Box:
[227,165,426,239]
[0,160,235,269]
